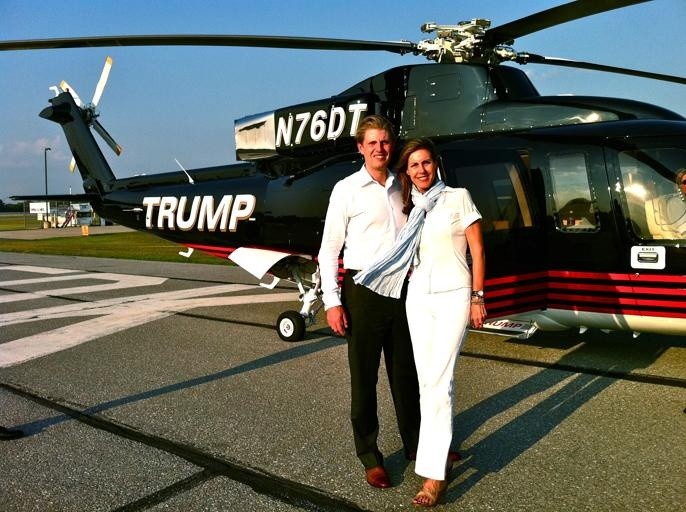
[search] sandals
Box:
[413,478,445,505]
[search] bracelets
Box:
[469,299,486,306]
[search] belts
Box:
[366,467,390,489]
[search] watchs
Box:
[472,289,485,297]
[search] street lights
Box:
[44,148,51,222]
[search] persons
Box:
[316,114,462,488]
[352,135,490,508]
[667,168,686,239]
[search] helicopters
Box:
[0,0,686,342]
